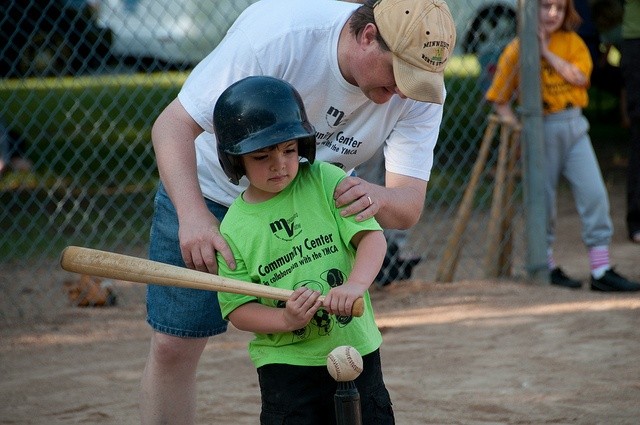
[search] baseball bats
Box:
[60,246,365,317]
[500,123,523,277]
[437,113,500,283]
[485,116,516,278]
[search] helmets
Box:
[213,75,316,186]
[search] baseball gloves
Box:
[64,271,119,308]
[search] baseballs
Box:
[326,346,363,382]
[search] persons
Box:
[487,1,639,296]
[621,3,640,246]
[209,74,398,423]
[144,1,457,421]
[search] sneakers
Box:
[551,268,581,288]
[591,267,640,291]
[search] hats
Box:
[372,0,456,105]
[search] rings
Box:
[364,192,373,207]
